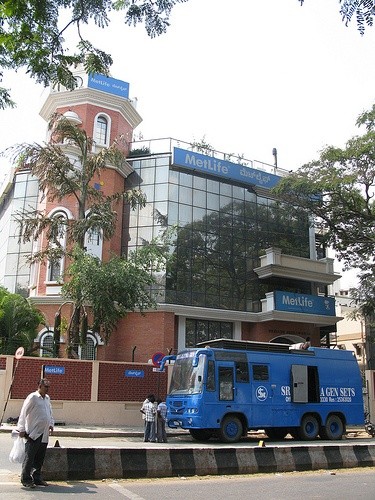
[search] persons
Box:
[139,398,157,443]
[157,398,169,443]
[142,394,156,440]
[16,379,55,489]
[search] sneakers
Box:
[34,481,48,486]
[21,481,36,487]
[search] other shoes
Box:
[144,440,168,443]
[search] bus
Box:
[160,338,365,442]
